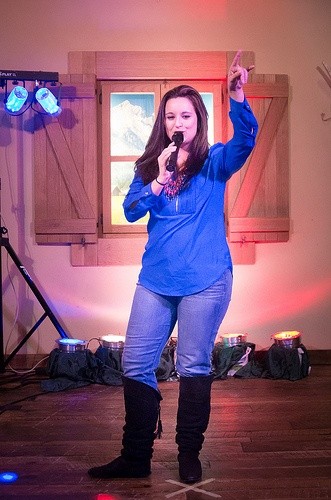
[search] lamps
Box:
[3,79,28,113]
[220,333,252,366]
[55,338,86,352]
[100,333,125,350]
[269,330,304,349]
[35,81,63,117]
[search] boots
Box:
[87,374,163,479]
[174,374,214,479]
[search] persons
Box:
[86,49,261,484]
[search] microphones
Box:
[165,132,184,172]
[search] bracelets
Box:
[156,177,166,186]
